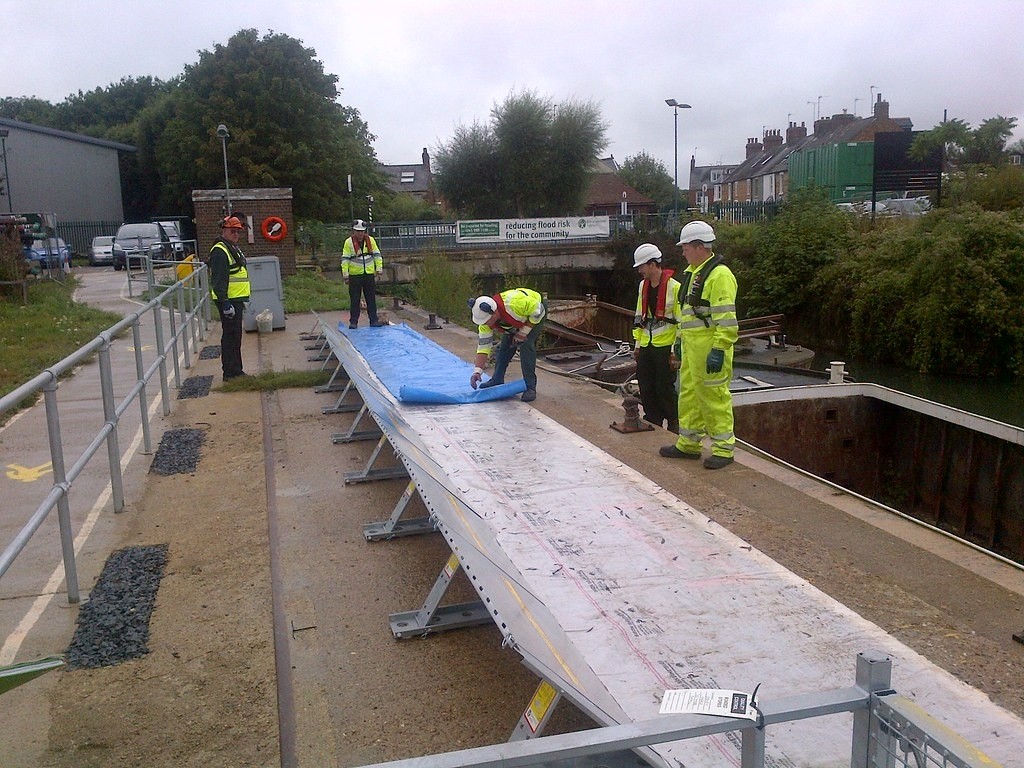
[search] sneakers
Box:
[522,387,535,401]
[703,454,733,469]
[371,321,382,327]
[349,323,357,329]
[479,378,504,388]
[659,444,701,459]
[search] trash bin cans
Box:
[243,256,287,332]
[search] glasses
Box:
[230,230,240,234]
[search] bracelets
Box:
[472,366,484,375]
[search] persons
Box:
[631,243,682,435]
[468,288,547,400]
[209,216,257,382]
[657,220,739,470]
[342,219,383,328]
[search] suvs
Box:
[112,221,174,271]
[151,220,188,261]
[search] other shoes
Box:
[223,373,256,382]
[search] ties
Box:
[496,320,506,327]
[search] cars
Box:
[836,190,937,220]
[23,236,72,270]
[87,235,115,266]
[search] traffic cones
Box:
[62,258,71,276]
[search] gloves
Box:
[706,349,724,374]
[377,271,383,279]
[470,373,482,390]
[512,332,527,345]
[242,299,251,313]
[674,337,681,361]
[633,348,639,363]
[669,353,680,371]
[223,305,235,319]
[343,277,350,284]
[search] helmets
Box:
[471,295,497,325]
[676,221,716,248]
[222,216,242,229]
[353,219,367,231]
[632,243,663,268]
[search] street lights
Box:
[216,123,232,216]
[665,98,692,216]
[0,129,12,213]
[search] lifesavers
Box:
[261,216,287,242]
